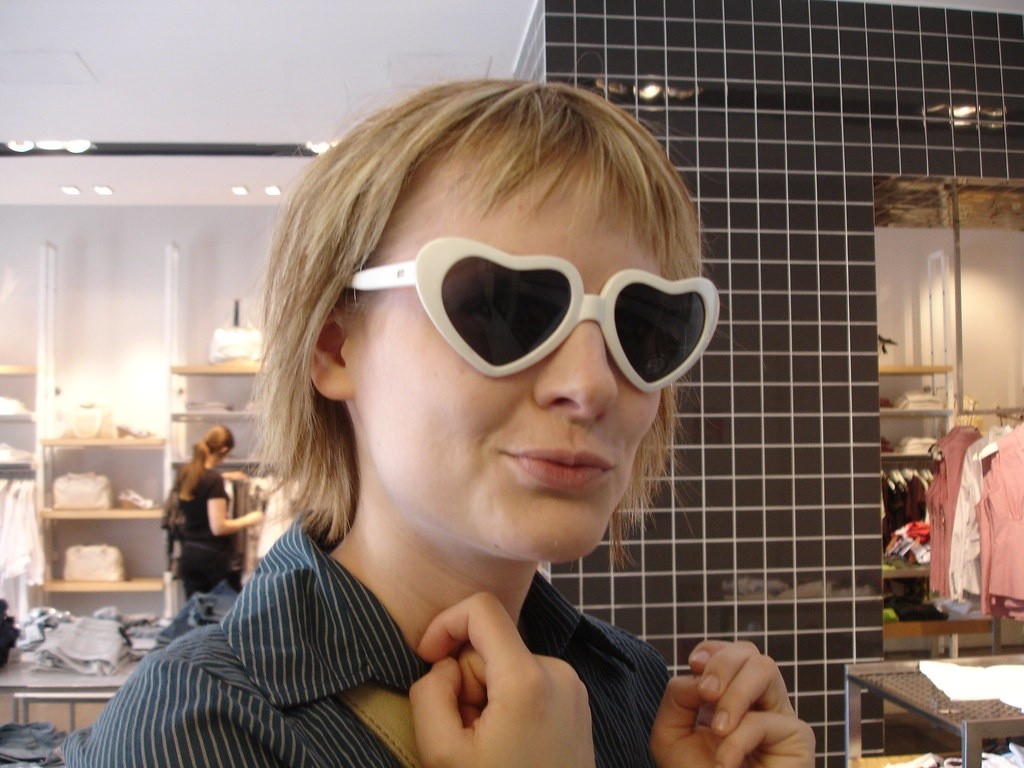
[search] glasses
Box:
[342,236,720,395]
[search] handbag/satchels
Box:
[63,544,125,582]
[52,471,113,510]
[61,401,118,439]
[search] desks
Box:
[0,660,140,738]
[845,654,1024,768]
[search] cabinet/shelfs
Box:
[878,368,952,625]
[171,361,281,618]
[34,435,172,621]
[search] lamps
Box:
[209,301,262,368]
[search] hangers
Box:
[951,405,1024,431]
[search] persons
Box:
[177,425,264,625]
[60,78,816,768]
